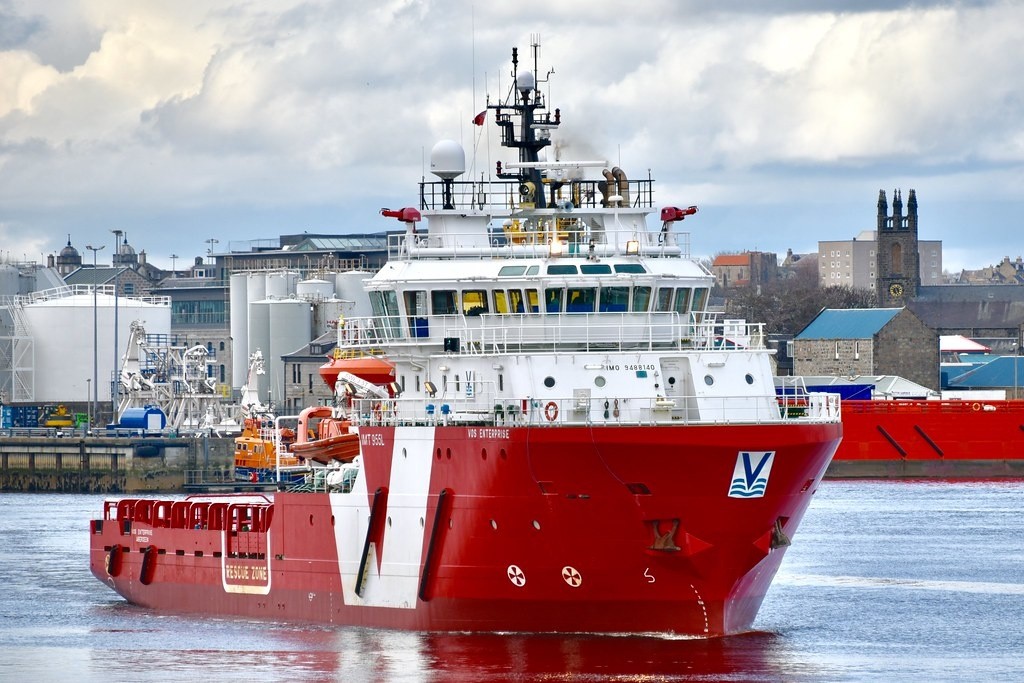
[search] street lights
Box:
[111,229,123,426]
[204,239,219,277]
[206,249,211,277]
[85,244,106,426]
[169,254,178,279]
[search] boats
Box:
[287,406,361,467]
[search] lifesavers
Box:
[544,402,557,420]
[973,403,980,411]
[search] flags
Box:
[471,110,487,126]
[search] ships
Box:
[88,1,844,641]
